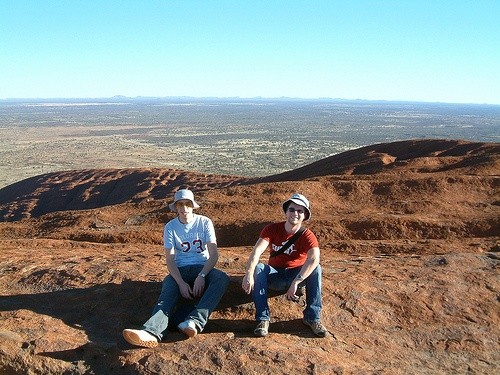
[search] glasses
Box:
[288,206,305,213]
[177,201,192,207]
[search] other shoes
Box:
[254,317,270,337]
[303,316,328,337]
[178,319,197,338]
[123,329,159,349]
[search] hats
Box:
[282,194,311,222]
[169,189,200,212]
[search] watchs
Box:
[198,273,206,278]
[296,274,304,282]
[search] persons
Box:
[242,194,329,337]
[123,189,229,349]
